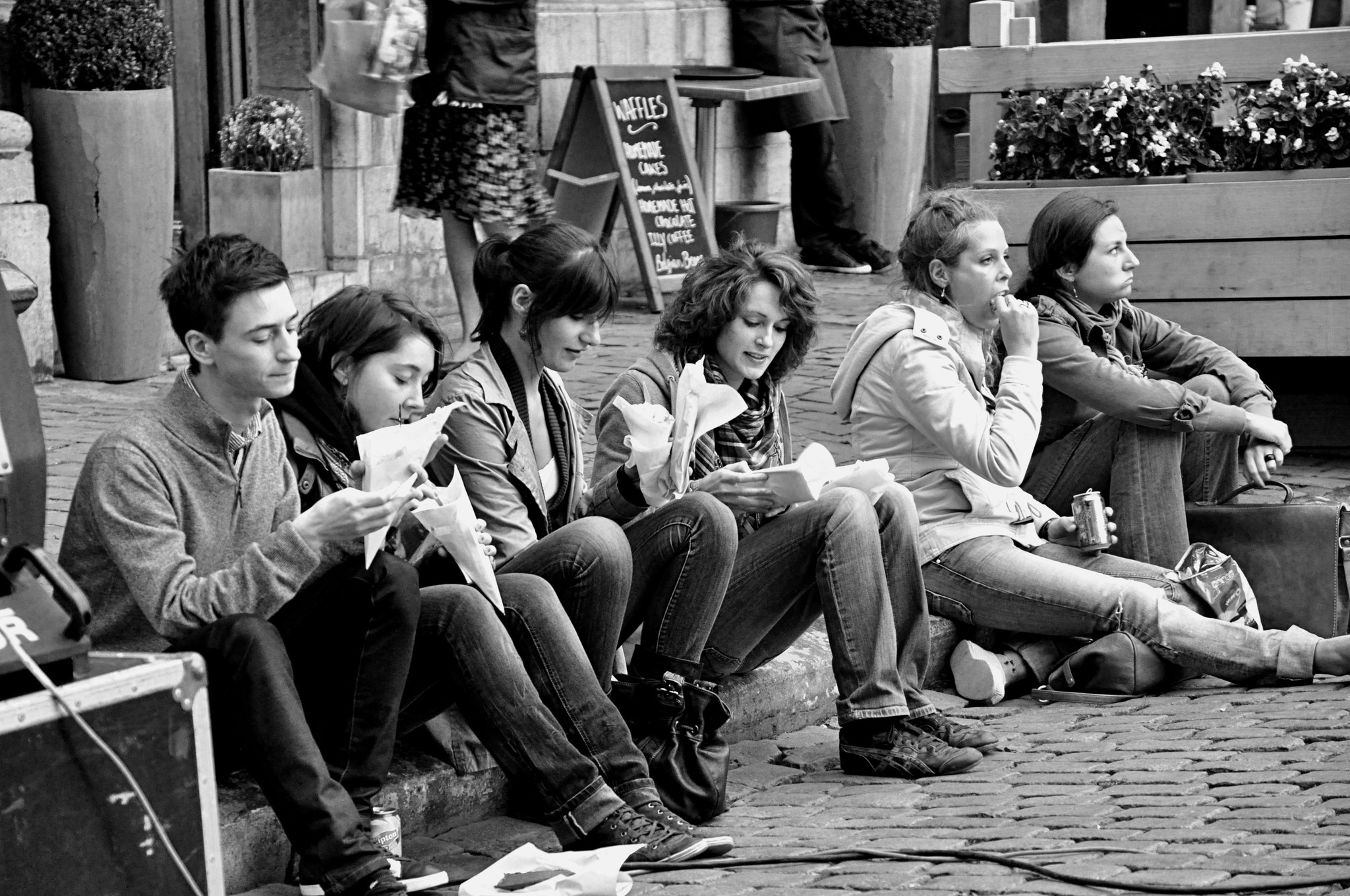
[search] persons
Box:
[267,286,734,866]
[386,0,558,381]
[421,220,740,699]
[829,185,1350,706]
[53,233,421,896]
[984,189,1294,573]
[731,0,900,274]
[591,238,999,779]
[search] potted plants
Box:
[11,0,178,382]
[824,0,940,252]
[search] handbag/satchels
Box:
[1032,631,1206,703]
[613,672,729,825]
[1185,480,1349,637]
[306,0,431,118]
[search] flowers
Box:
[989,53,1350,177]
[221,94,309,171]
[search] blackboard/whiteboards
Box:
[542,65,720,292]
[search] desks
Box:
[666,64,823,233]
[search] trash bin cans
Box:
[716,200,781,251]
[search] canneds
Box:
[370,807,401,877]
[1071,488,1112,552]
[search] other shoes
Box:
[437,359,466,379]
[951,639,1007,706]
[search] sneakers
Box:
[563,807,706,868]
[632,802,735,857]
[839,722,983,777]
[299,833,449,896]
[913,714,998,752]
[350,868,406,896]
[800,241,871,274]
[835,232,900,273]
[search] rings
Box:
[1019,300,1028,308]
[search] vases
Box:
[964,166,1350,357]
